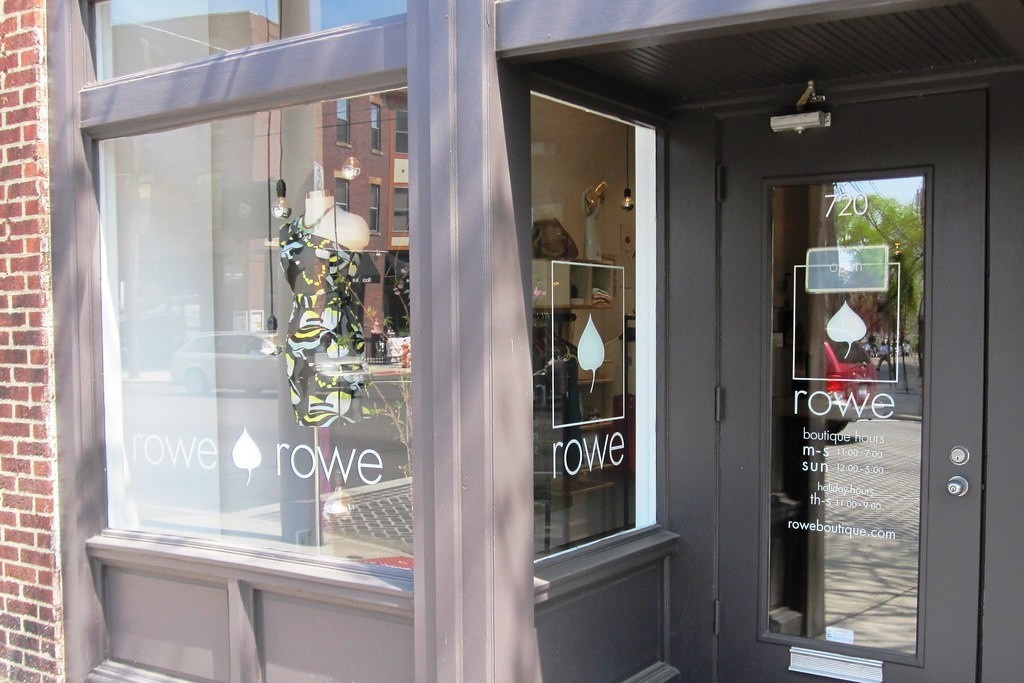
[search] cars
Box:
[170,331,371,401]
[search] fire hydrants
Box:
[401,342,412,368]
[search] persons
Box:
[864,338,914,372]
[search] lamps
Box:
[586,181,609,210]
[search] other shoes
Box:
[876,368,880,370]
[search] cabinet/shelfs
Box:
[532,258,628,545]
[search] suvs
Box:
[825,338,884,435]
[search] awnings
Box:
[330,252,381,283]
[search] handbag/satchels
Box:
[532,218,579,261]
